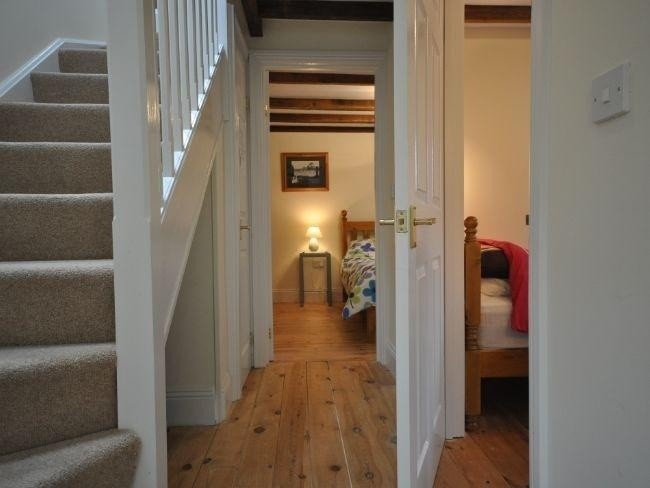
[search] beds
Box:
[466,216,528,433]
[340,208,375,342]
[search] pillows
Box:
[481,276,511,297]
[481,244,509,280]
[344,239,375,260]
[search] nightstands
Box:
[299,252,333,307]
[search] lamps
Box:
[306,226,324,251]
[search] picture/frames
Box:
[279,152,330,192]
